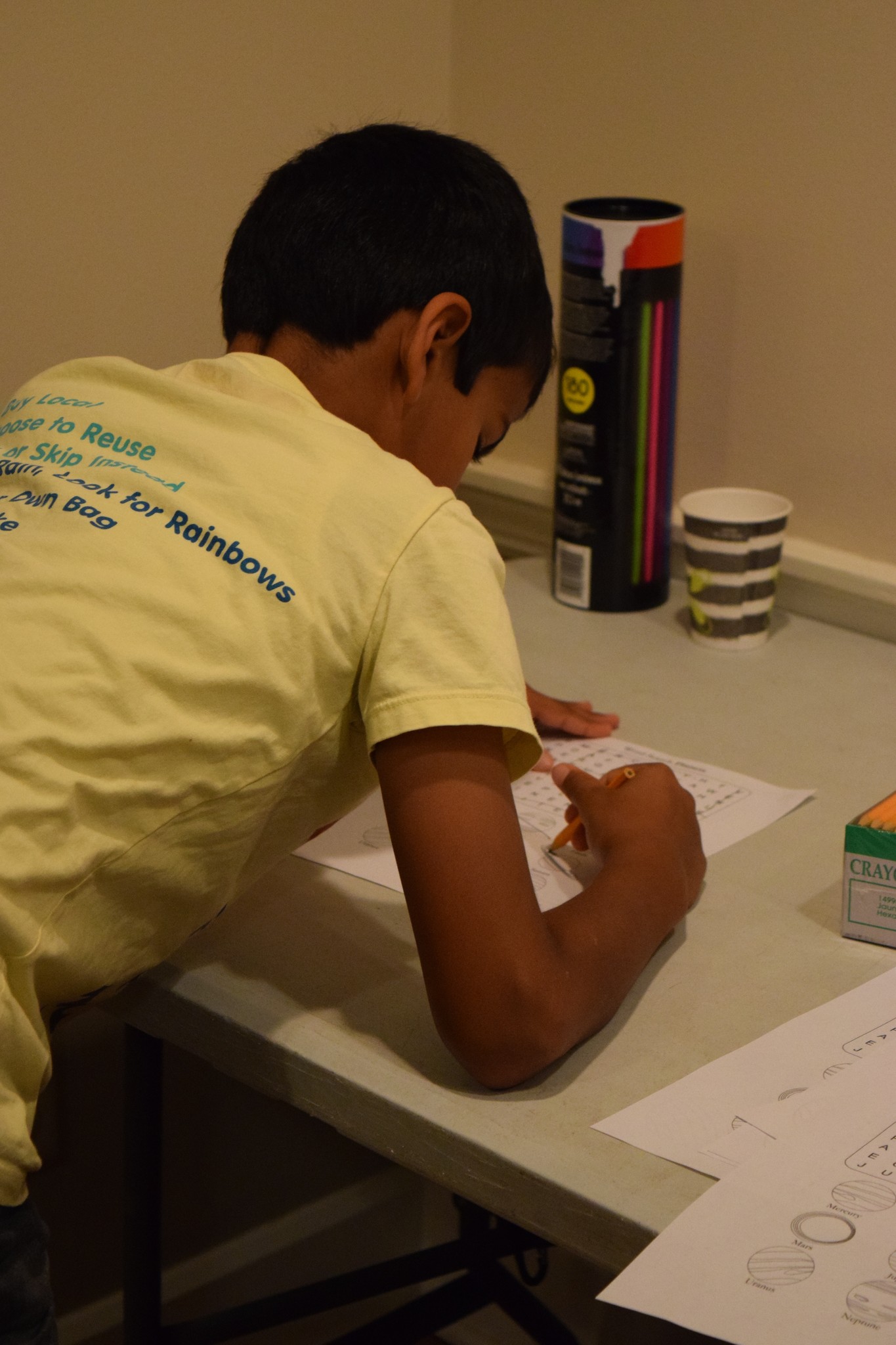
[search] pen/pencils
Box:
[548,768,635,853]
[857,792,896,831]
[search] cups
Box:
[677,487,794,652]
[551,198,685,613]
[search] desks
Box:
[82,554,896,1345]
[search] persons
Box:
[0,123,706,1343]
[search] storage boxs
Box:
[840,791,896,950]
